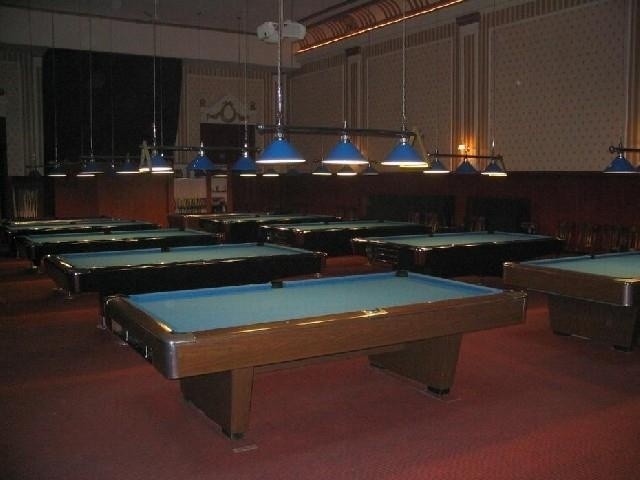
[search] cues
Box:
[177,191,201,214]
[12,185,39,220]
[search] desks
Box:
[38,240,329,346]
[497,249,638,357]
[6,212,164,272]
[18,225,221,301]
[95,265,529,435]
[45,2,176,178]
[345,228,572,280]
[257,218,435,279]
[179,213,344,244]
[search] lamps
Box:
[422,150,509,182]
[254,0,308,167]
[379,1,427,170]
[214,171,283,178]
[230,3,257,171]
[185,141,218,171]
[601,145,639,174]
[319,120,370,167]
[309,164,380,178]
